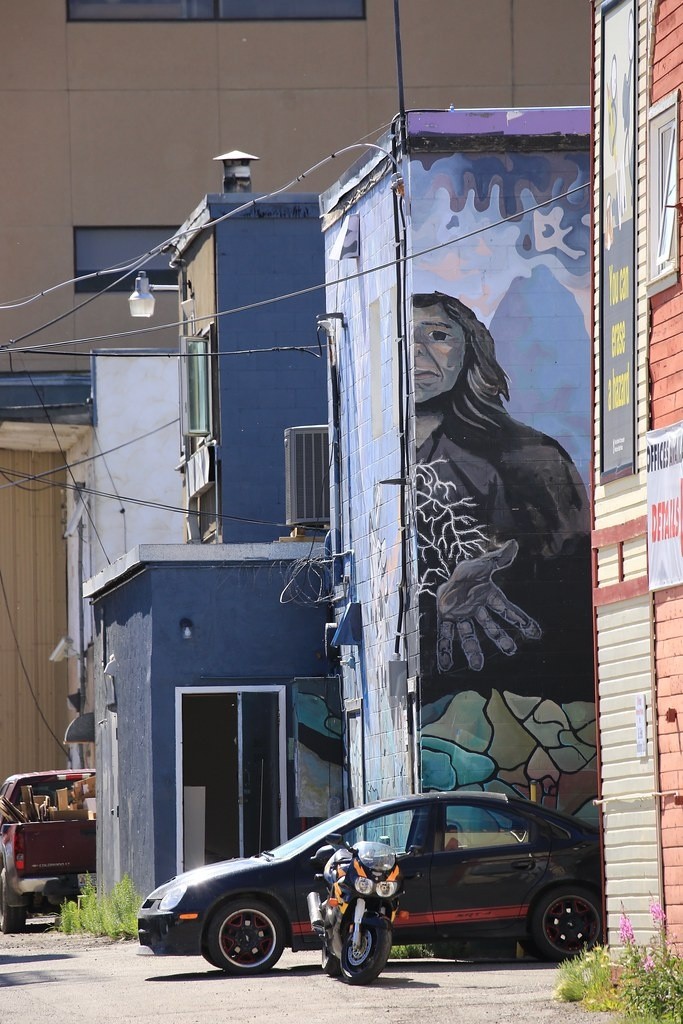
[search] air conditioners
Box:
[283,423,327,522]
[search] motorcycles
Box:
[307,834,426,985]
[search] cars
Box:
[138,791,608,975]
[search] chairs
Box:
[32,790,53,806]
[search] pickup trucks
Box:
[0,768,96,933]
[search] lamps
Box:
[128,270,179,317]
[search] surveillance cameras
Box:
[49,637,72,661]
